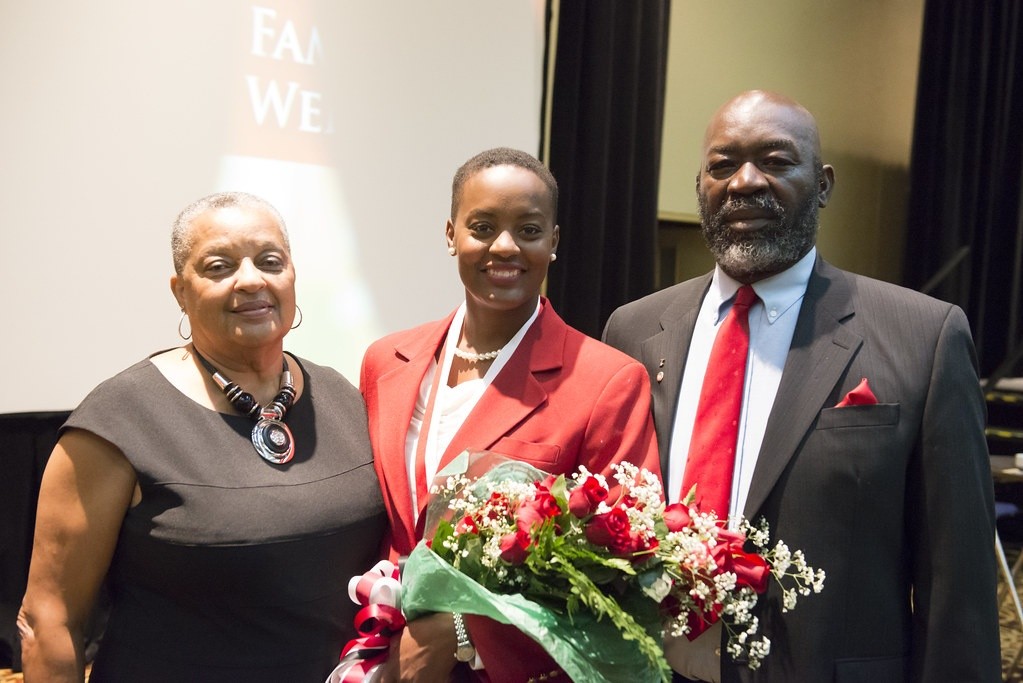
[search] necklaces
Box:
[454,348,502,361]
[193,345,298,465]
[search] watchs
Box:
[453,612,475,662]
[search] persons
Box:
[16,191,387,683]
[602,90,1003,683]
[360,148,664,683]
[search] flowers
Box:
[394,446,827,683]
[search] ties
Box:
[672,284,757,641]
